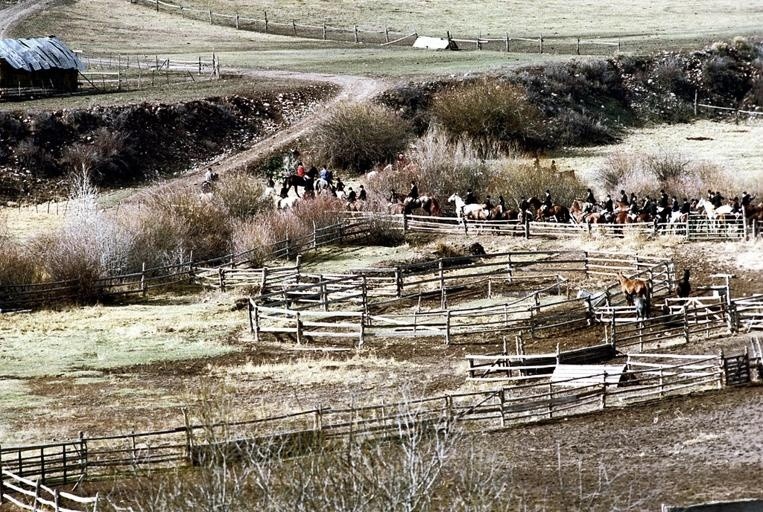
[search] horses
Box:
[676,267,691,309]
[389,188,439,217]
[612,198,645,228]
[526,195,569,223]
[490,204,503,220]
[501,207,515,220]
[695,196,732,231]
[316,171,334,194]
[447,191,482,225]
[569,200,602,224]
[616,271,647,312]
[283,165,318,200]
